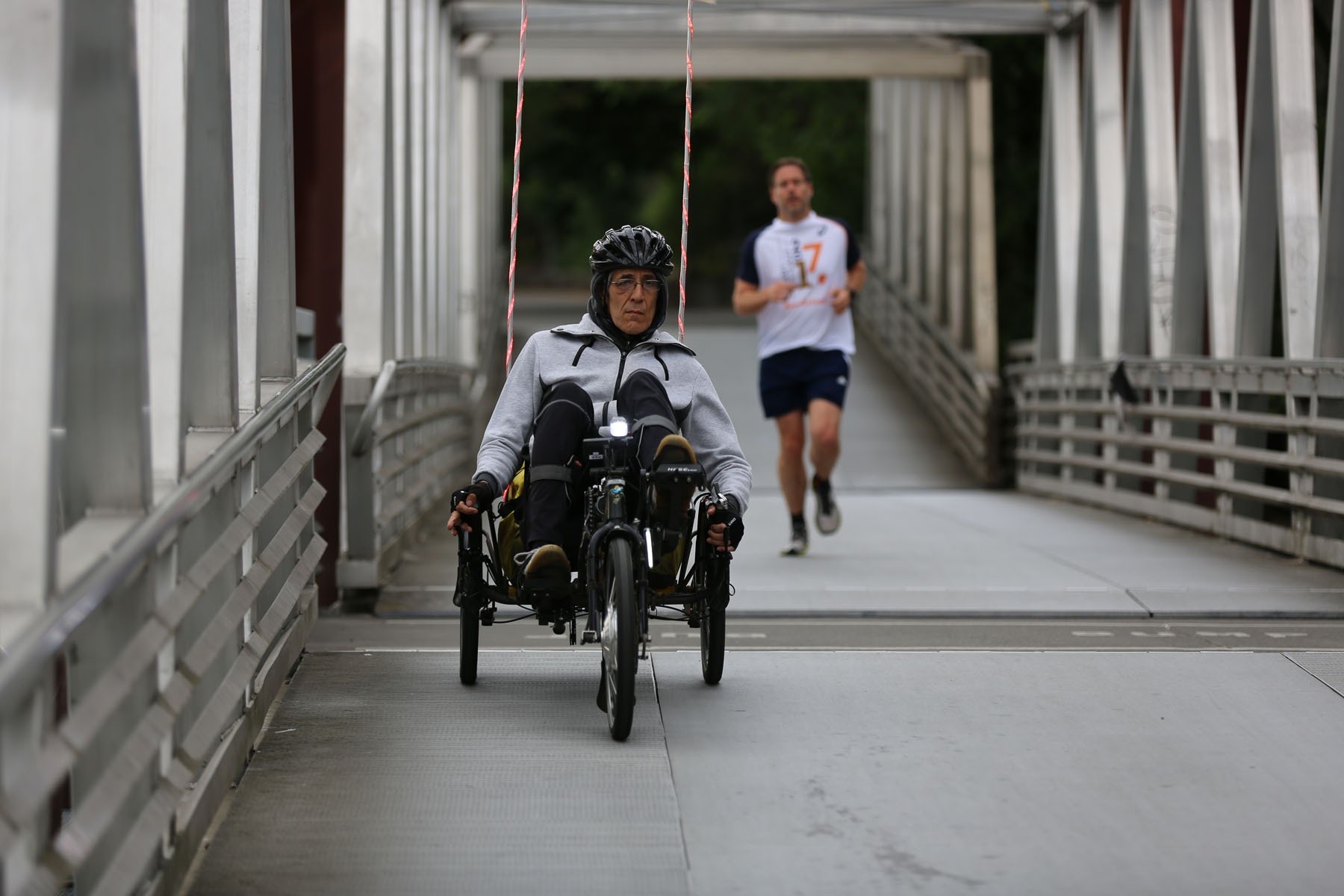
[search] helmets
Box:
[589,225,674,277]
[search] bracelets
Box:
[848,286,855,298]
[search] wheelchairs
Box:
[453,428,734,742]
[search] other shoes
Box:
[514,544,572,590]
[788,525,807,553]
[647,435,697,533]
[812,474,840,534]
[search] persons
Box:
[732,156,865,555]
[447,225,754,622]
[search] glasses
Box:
[611,278,663,292]
